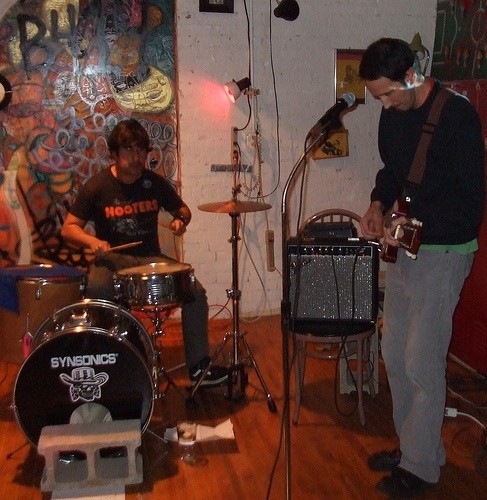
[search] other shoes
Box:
[188,365,229,387]
[367,447,403,472]
[374,466,439,496]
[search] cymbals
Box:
[197,201,272,213]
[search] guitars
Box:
[378,200,423,264]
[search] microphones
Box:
[304,90,356,140]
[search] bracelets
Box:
[178,215,188,223]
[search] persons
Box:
[61,119,230,386]
[359,38,484,496]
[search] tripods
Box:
[189,213,276,410]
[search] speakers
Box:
[285,236,381,326]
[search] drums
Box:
[14,299,157,465]
[0,264,83,365]
[114,263,194,307]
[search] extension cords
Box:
[265,230,275,271]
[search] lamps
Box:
[223,77,251,102]
[274,0,299,21]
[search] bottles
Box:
[176,414,197,466]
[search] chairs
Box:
[292,208,377,426]
